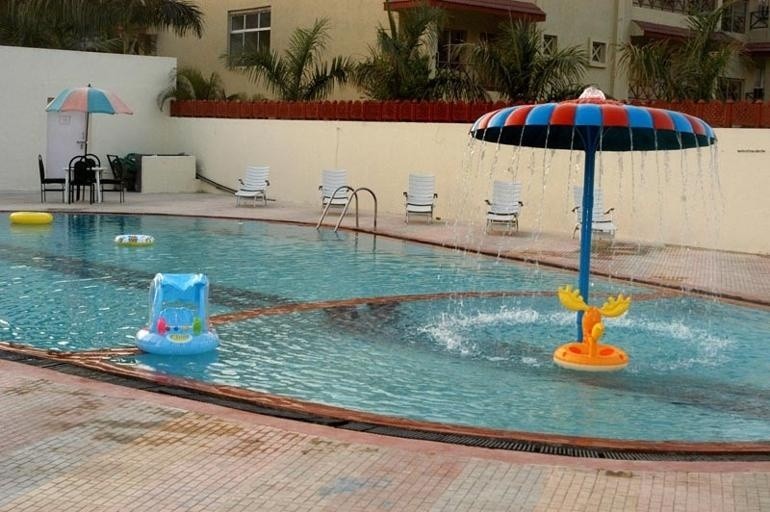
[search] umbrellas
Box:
[46,85,133,203]
[468,86,718,344]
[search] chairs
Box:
[317,166,352,216]
[571,186,618,248]
[403,171,441,227]
[233,163,273,209]
[480,177,526,237]
[34,154,136,207]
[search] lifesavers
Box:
[10,211,53,224]
[114,234,155,246]
[554,285,633,369]
[136,274,218,353]
